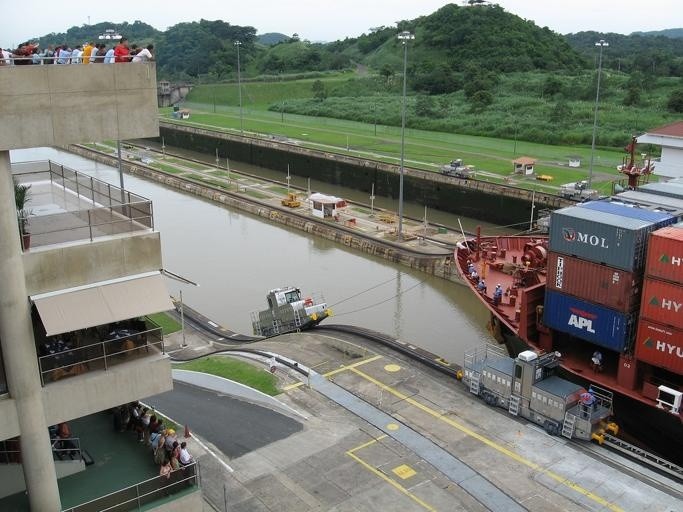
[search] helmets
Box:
[496,284,500,288]
[167,429,175,435]
[470,264,473,266]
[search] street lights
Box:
[232,40,243,133]
[395,26,414,238]
[588,37,610,188]
[97,26,129,219]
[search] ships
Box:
[448,233,682,467]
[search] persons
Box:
[109,400,194,478]
[466,256,503,308]
[579,388,601,418]
[590,347,603,378]
[0,37,156,67]
[56,423,77,461]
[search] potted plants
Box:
[16,209,35,251]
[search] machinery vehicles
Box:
[459,344,622,452]
[281,192,300,209]
[250,287,332,338]
[439,157,476,181]
[557,180,596,202]
